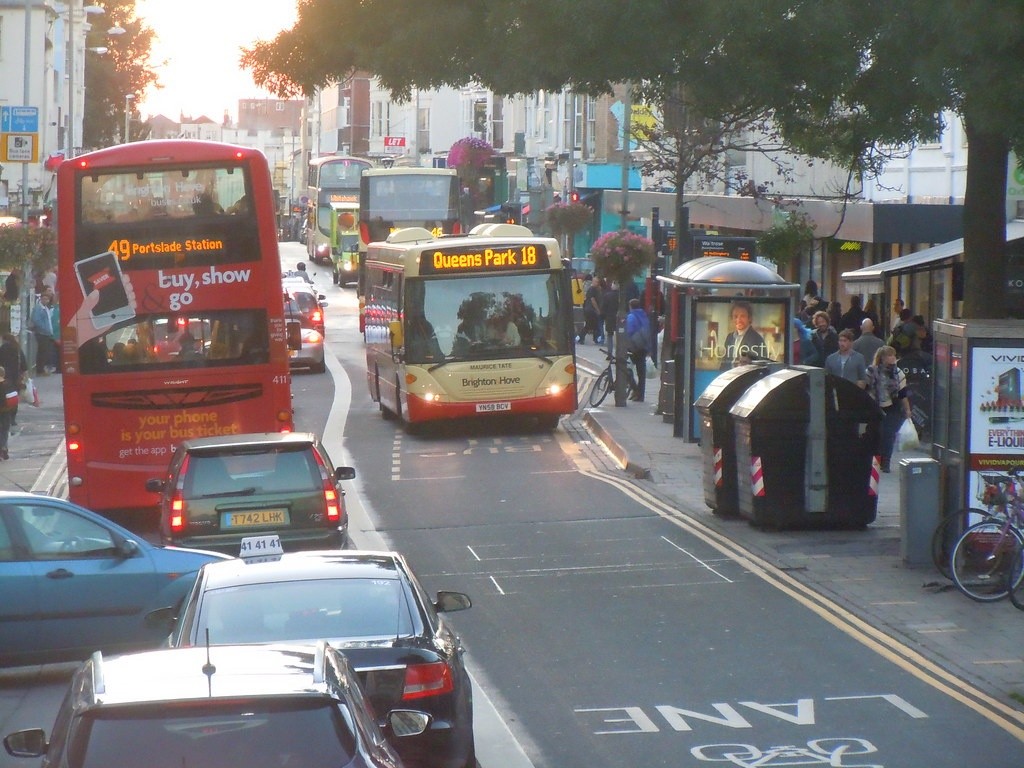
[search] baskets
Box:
[977,471,1012,505]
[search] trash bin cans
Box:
[692,362,771,513]
[898,457,946,564]
[662,360,675,424]
[726,364,888,532]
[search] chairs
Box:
[275,453,306,477]
[193,456,232,487]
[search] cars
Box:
[145,431,357,555]
[569,257,596,279]
[281,276,330,373]
[0,491,236,668]
[2,638,433,768]
[143,551,474,767]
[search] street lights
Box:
[125,94,135,142]
[279,126,295,241]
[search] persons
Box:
[717,300,768,370]
[0,330,28,462]
[27,285,63,376]
[452,218,465,239]
[626,296,652,403]
[642,277,666,370]
[792,279,935,475]
[107,317,265,365]
[294,261,310,285]
[487,304,521,348]
[112,189,224,224]
[577,277,640,362]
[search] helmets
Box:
[297,262,306,271]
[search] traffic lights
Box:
[571,193,580,203]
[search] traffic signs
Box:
[10,105,40,132]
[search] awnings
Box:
[473,191,596,221]
[839,216,1024,296]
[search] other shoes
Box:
[629,394,645,401]
[593,338,599,344]
[880,459,890,473]
[0,449,9,460]
[578,335,586,344]
[36,371,50,376]
[11,420,17,426]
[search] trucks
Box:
[329,202,360,288]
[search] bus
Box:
[308,156,377,263]
[356,165,465,300]
[364,222,579,429]
[55,139,296,512]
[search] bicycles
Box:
[931,466,1024,611]
[589,348,634,408]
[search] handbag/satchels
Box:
[23,378,40,407]
[897,417,919,452]
[628,313,653,353]
